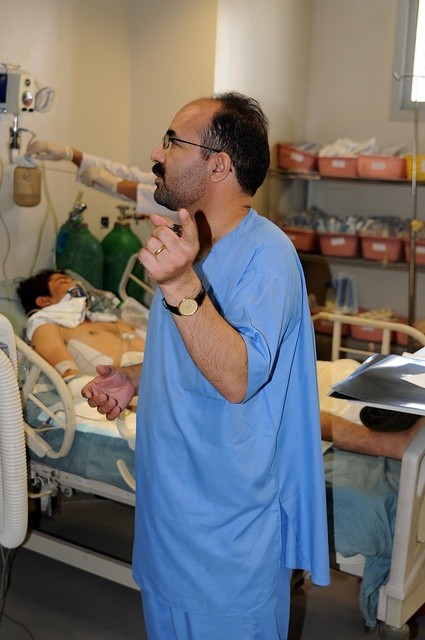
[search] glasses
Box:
[163,134,232,172]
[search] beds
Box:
[0,252,425,638]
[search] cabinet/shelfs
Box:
[267,169,425,361]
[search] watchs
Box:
[161,284,209,317]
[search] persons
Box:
[27,139,180,307]
[81,91,331,640]
[16,267,425,462]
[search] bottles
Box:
[14,157,42,208]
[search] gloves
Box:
[79,165,125,193]
[27,140,73,162]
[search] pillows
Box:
[0,268,120,346]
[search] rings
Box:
[155,246,166,256]
